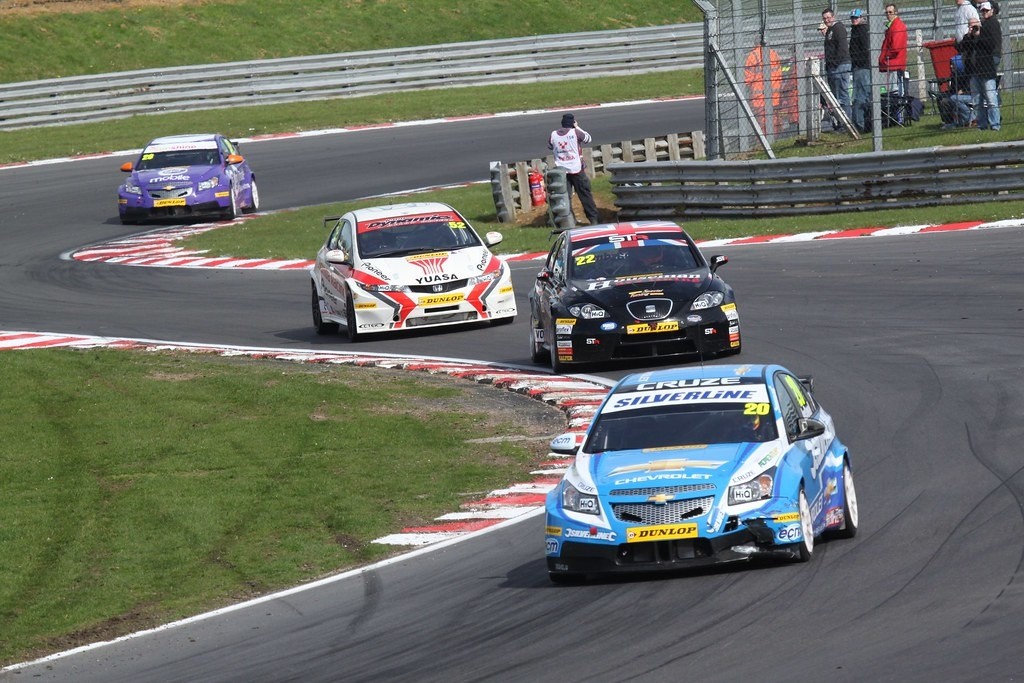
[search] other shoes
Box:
[940,124,952,129]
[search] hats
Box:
[562,114,575,125]
[850,8,863,17]
[980,1,992,10]
[817,21,828,31]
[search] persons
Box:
[878,3,908,127]
[937,0,1002,131]
[546,113,602,225]
[745,35,783,141]
[783,42,798,125]
[848,7,872,133]
[817,9,852,133]
[740,414,762,441]
[631,245,663,263]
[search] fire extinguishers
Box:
[528,166,546,206]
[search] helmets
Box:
[636,245,664,267]
[734,412,760,435]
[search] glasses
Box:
[823,16,833,21]
[886,11,894,13]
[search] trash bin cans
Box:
[922,38,959,91]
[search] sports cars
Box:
[117,133,260,225]
[529,219,743,370]
[310,201,518,343]
[544,362,860,585]
